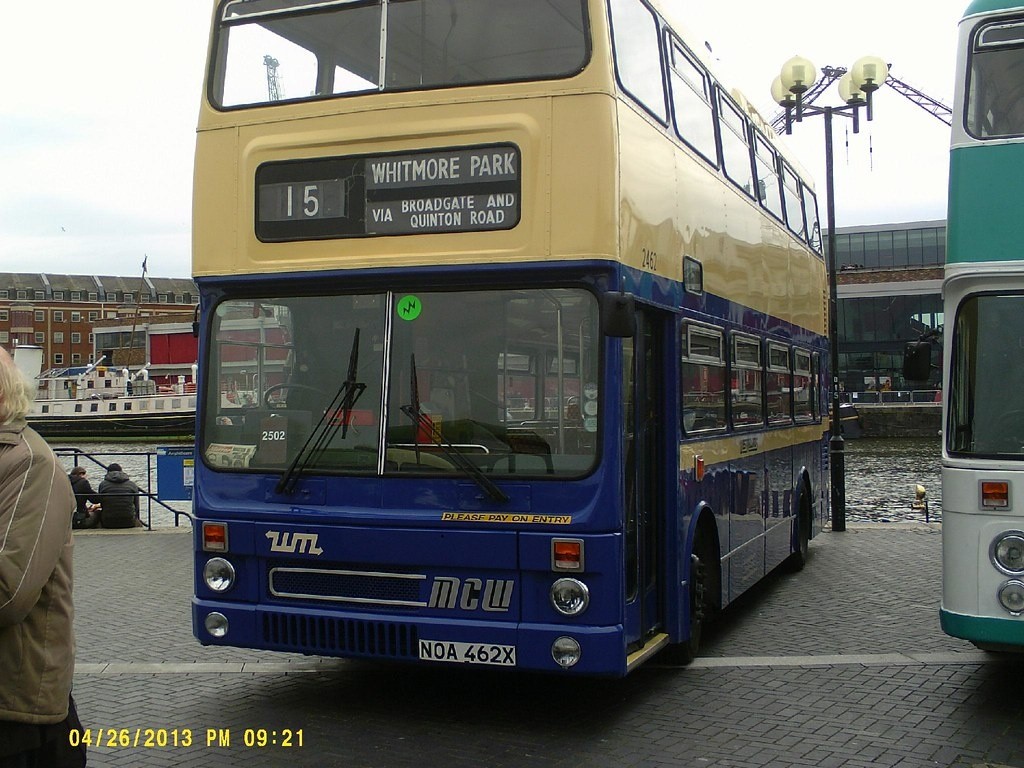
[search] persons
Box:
[0,344,87,768]
[67,463,143,529]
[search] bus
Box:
[939,0,1024,657]
[187,0,832,681]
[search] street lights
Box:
[770,57,889,531]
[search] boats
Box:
[23,366,279,443]
